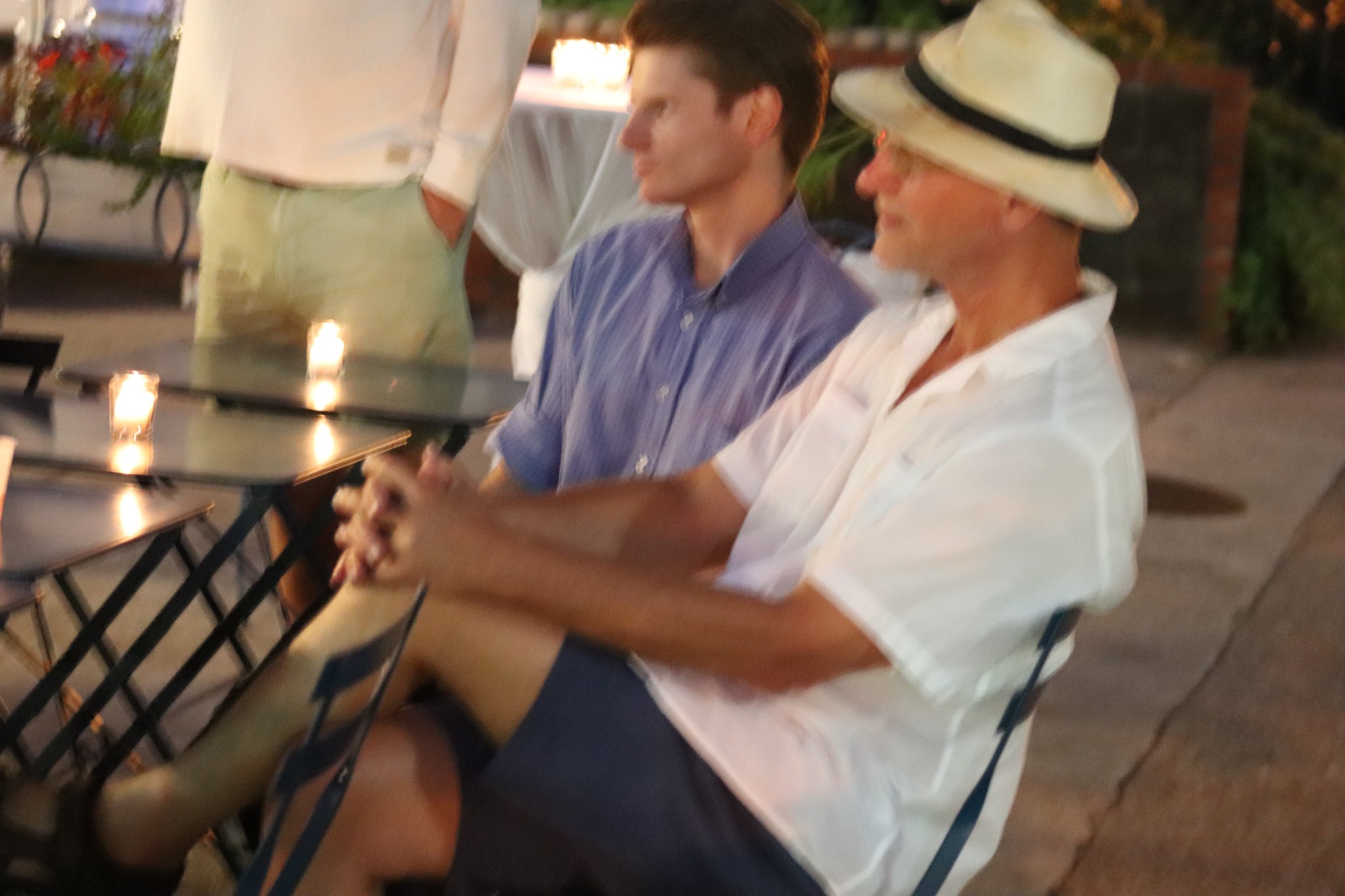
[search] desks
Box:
[0,334,540,798]
[467,64,688,379]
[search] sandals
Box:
[0,767,190,896]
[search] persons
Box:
[1,0,1148,895]
[155,1,549,653]
[187,0,910,896]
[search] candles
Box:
[104,370,159,464]
[303,309,345,375]
[550,34,633,86]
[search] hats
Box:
[829,0,1137,237]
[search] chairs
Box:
[232,576,436,896]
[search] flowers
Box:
[0,29,211,162]
